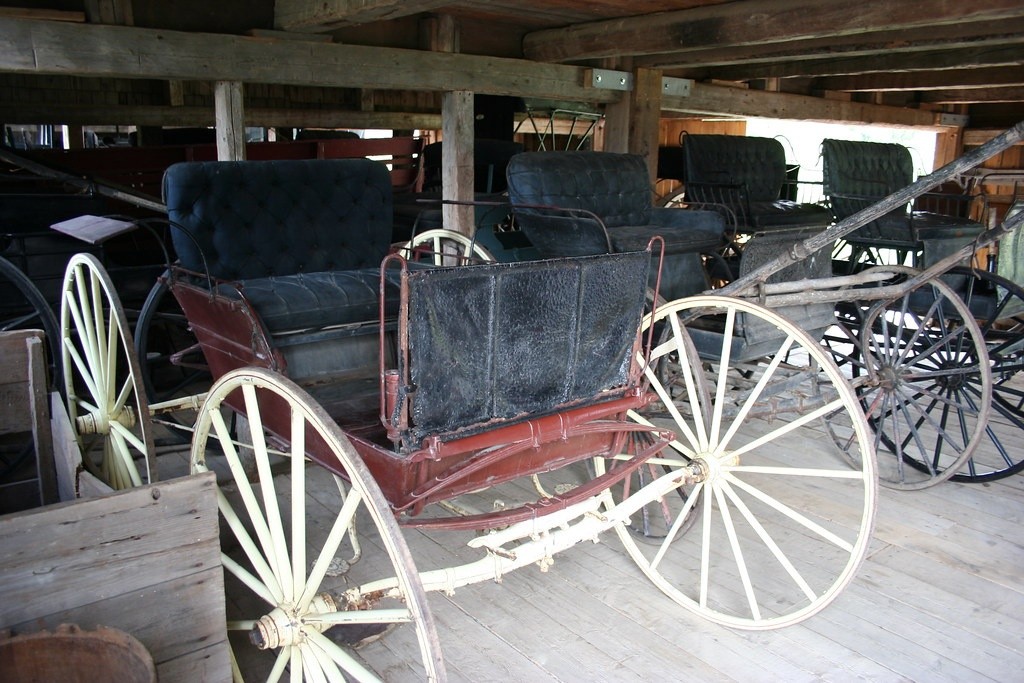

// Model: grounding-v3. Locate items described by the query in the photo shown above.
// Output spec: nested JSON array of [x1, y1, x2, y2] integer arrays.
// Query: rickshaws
[[55, 131, 1024, 682]]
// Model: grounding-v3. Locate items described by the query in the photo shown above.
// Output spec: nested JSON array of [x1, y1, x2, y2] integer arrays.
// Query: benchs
[[822, 138, 991, 244], [679, 135, 835, 233], [507, 153, 737, 256], [159, 156, 407, 401], [0, 174, 177, 318]]
